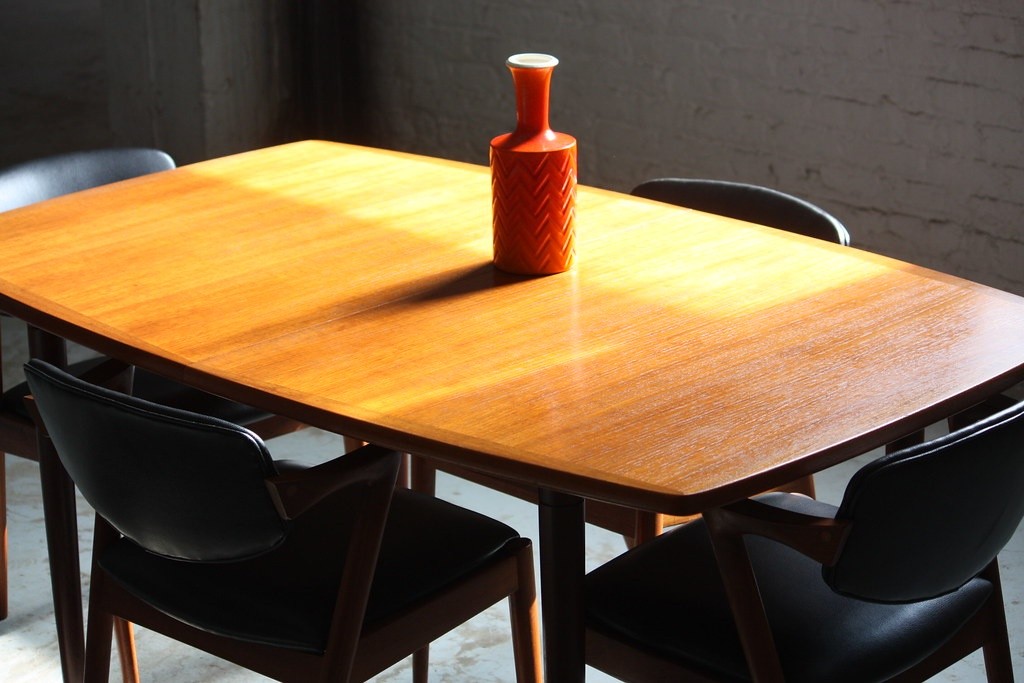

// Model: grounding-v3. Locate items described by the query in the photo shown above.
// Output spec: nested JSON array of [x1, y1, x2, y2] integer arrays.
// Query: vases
[[489, 52, 578, 274]]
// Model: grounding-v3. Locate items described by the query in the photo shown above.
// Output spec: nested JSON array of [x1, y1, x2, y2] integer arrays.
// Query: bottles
[[488, 51, 579, 273]]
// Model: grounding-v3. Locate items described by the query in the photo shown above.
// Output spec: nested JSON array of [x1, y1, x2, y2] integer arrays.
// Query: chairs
[[582, 395, 1024, 682], [0, 149, 407, 682], [413, 179, 853, 683], [23, 357, 542, 683]]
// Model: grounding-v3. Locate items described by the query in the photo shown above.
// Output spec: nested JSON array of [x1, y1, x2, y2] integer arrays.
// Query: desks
[[0, 138, 1024, 683]]
